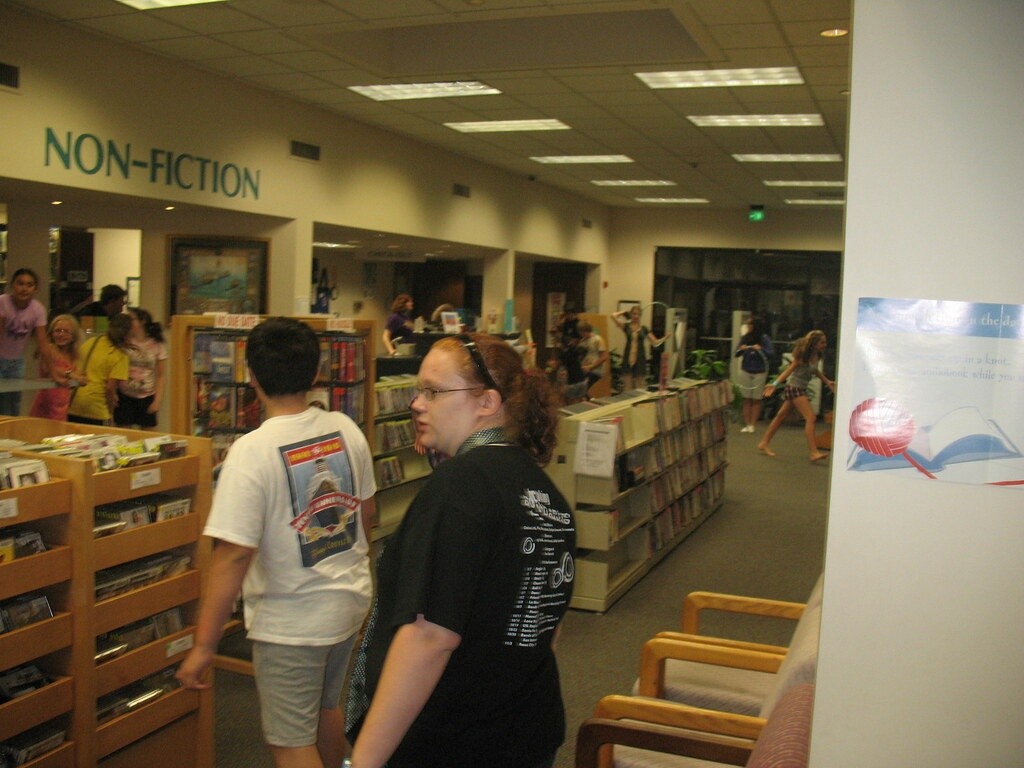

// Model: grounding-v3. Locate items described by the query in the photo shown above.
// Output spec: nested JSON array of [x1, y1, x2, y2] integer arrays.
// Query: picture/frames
[[171, 237, 270, 315]]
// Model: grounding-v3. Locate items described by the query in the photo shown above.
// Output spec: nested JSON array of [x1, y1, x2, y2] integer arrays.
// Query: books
[[372, 374, 428, 492], [0, 608, 182, 706], [1, 429, 190, 489], [194, 328, 369, 615], [557, 378, 732, 555], [0, 550, 190, 633], [0, 665, 185, 768], [0, 496, 193, 563]]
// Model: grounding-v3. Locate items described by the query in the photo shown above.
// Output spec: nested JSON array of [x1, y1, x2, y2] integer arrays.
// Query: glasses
[[414, 387, 505, 402], [51, 327, 73, 336]]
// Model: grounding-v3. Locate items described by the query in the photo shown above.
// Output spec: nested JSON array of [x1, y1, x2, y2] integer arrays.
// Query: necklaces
[[456, 426, 520, 457]]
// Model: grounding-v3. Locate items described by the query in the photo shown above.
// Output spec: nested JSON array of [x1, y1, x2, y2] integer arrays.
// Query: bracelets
[[341, 758, 353, 768], [772, 381, 779, 387], [65, 369, 72, 379]]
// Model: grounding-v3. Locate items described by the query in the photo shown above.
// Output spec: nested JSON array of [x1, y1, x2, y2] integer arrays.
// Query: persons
[[178, 316, 376, 768], [543, 305, 672, 406], [758, 330, 835, 463], [0, 266, 167, 431], [343, 332, 577, 768], [382, 294, 415, 356], [734, 315, 775, 434]]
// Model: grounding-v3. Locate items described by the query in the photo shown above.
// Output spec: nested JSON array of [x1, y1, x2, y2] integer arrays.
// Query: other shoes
[[741, 425, 754, 432]]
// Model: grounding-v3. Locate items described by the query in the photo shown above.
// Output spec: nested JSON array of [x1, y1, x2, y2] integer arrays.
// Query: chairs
[[574, 571, 823, 768]]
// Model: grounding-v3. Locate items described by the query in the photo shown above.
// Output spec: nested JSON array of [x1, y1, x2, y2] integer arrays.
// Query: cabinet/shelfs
[[1, 315, 732, 768]]
